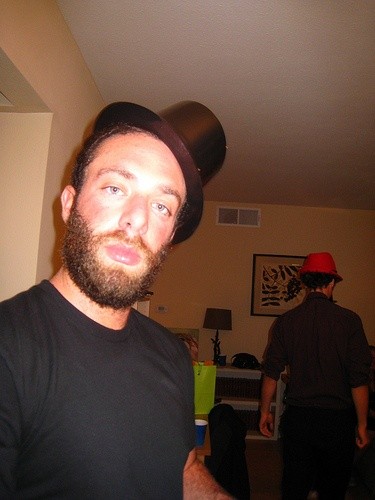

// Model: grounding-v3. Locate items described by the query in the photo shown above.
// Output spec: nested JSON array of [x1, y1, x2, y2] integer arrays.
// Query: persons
[[0, 121, 241, 500], [258, 253, 370, 500]]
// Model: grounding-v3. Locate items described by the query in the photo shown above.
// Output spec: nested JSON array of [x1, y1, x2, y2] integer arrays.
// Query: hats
[[92, 100, 227, 244], [297, 251, 343, 284]]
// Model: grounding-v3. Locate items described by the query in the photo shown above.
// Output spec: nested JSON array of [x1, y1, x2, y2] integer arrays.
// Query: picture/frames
[[250, 253, 333, 317]]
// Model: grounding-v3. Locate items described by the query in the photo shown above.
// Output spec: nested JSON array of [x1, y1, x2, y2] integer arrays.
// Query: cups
[[194, 419, 208, 446], [217, 354, 226, 367]]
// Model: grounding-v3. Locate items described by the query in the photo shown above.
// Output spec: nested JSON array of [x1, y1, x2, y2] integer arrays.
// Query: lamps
[[202, 307, 232, 365]]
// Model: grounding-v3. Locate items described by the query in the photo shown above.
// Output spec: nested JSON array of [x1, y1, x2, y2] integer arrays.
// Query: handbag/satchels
[[190, 358, 217, 415]]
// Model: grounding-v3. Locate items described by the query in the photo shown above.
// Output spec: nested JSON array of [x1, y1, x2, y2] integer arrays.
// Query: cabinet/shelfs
[[215, 365, 286, 441]]
